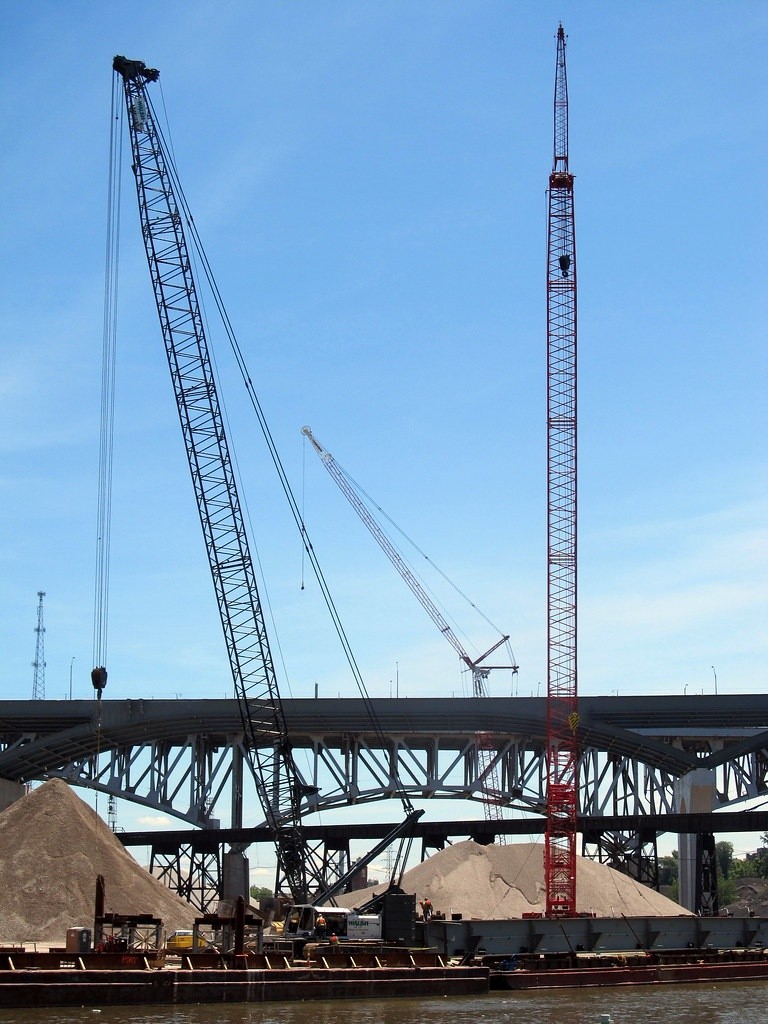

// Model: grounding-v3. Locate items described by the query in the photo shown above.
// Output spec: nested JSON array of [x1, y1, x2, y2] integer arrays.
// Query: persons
[[316, 914, 326, 940], [420, 898, 433, 921], [330, 932, 341, 953]]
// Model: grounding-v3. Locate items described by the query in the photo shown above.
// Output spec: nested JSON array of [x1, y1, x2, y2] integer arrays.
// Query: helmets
[[319, 914, 322, 917], [420, 902, 422, 904], [332, 933, 336, 936], [425, 897, 428, 900]]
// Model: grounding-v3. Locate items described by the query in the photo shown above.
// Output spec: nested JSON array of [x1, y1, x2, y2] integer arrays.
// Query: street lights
[[711, 665, 718, 696], [69, 656, 76, 701], [389, 680, 394, 699], [683, 684, 689, 697], [537, 682, 541, 699], [396, 662, 400, 698]]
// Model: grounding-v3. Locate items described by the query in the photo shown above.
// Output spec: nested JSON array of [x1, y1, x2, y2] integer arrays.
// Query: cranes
[[540, 18, 586, 914], [28, 590, 47, 700], [80, 49, 425, 907], [296, 422, 527, 841]]
